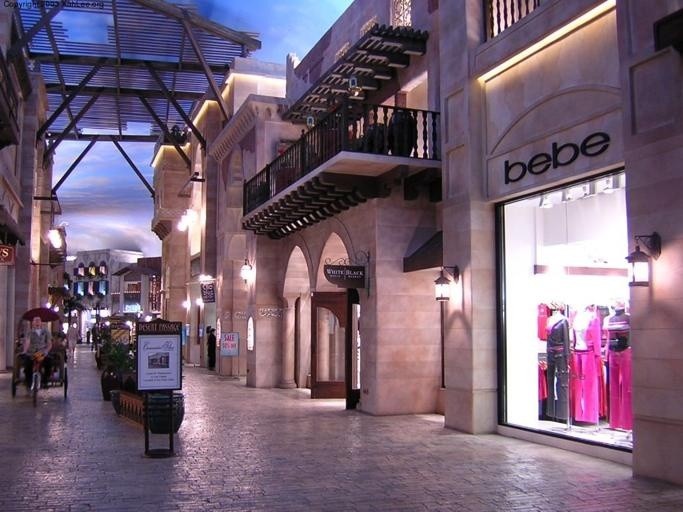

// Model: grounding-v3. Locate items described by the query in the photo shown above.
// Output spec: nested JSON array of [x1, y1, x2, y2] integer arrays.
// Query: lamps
[[626, 230, 661, 287], [434, 264, 460, 303]]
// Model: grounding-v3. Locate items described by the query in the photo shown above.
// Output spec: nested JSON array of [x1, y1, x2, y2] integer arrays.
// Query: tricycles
[[11, 308, 68, 407]]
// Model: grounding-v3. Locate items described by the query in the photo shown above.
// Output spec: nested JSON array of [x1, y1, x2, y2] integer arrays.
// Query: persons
[[605, 307, 635, 431], [67, 322, 81, 359], [571, 305, 605, 425], [21, 315, 54, 395], [90, 323, 101, 351], [209, 328, 216, 371], [543, 308, 570, 420]]
